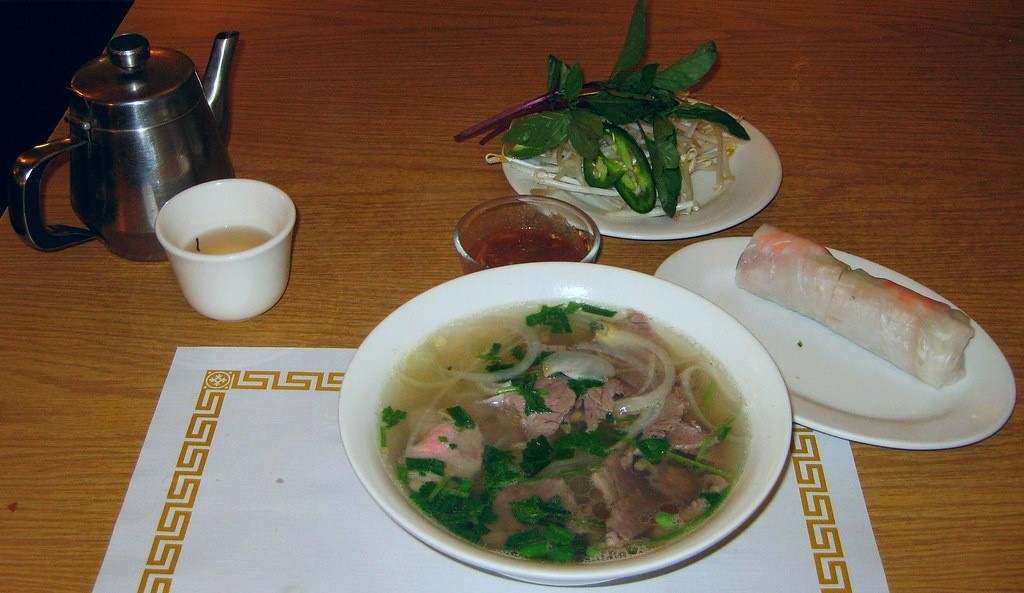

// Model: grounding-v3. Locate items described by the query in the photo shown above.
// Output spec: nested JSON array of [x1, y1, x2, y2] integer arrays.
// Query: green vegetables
[[379, 296, 742, 565], [454, 0, 751, 216]]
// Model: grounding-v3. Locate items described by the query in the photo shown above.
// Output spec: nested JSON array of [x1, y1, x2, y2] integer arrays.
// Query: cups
[[451, 192, 602, 275], [154, 179, 298, 321]]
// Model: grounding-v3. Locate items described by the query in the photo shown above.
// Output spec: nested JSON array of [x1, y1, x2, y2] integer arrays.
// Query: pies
[[732, 221, 974, 386]]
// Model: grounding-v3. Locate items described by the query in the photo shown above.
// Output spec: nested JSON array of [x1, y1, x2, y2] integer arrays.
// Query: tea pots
[[10, 30, 241, 262]]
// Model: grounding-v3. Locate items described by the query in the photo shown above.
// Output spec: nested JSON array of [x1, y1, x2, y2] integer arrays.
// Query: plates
[[502, 90, 783, 240], [653, 237, 1015, 451]]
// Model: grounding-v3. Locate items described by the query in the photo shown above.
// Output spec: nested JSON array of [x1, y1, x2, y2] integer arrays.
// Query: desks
[[0, 0, 1024, 593]]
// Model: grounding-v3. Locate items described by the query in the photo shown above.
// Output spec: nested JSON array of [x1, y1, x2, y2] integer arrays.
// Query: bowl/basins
[[339, 260, 793, 586]]
